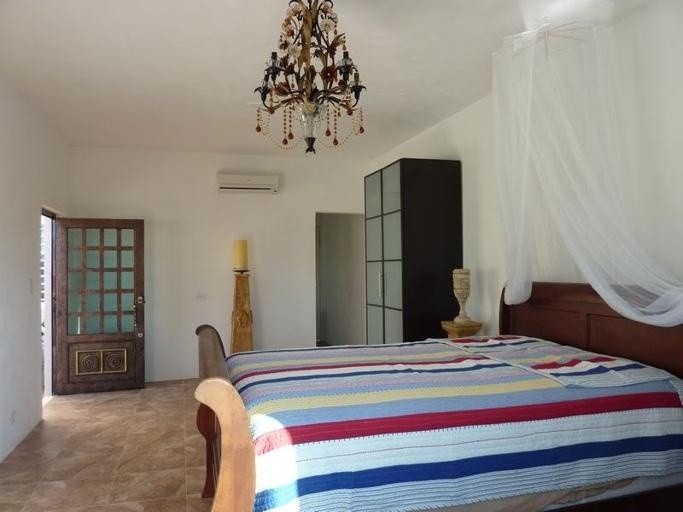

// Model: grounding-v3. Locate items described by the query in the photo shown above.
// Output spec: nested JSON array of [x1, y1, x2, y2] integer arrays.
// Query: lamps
[[452, 267, 472, 322], [252, 0, 366, 156]]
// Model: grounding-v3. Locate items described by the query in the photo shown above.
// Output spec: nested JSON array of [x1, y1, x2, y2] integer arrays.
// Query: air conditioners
[[215, 171, 280, 195]]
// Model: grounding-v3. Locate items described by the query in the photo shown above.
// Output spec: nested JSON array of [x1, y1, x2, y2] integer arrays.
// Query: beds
[[192, 280, 683, 512]]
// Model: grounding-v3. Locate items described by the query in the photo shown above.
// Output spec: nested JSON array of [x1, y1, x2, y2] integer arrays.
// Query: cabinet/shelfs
[[363, 157, 464, 346]]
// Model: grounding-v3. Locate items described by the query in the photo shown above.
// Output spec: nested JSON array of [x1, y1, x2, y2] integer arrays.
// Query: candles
[[234, 239, 249, 268]]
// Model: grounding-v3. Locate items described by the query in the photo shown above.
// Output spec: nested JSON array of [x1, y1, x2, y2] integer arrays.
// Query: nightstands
[[440, 320, 482, 339]]
